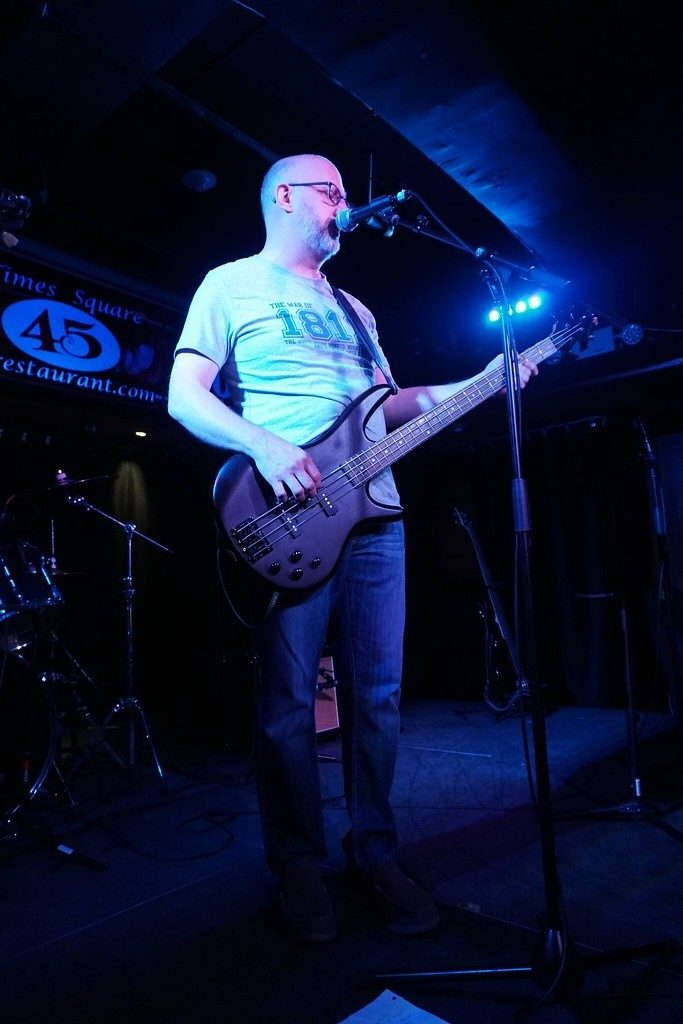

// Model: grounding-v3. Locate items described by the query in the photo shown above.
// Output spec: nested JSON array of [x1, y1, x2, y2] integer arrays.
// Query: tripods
[[360, 207, 682, 1023], [63, 488, 180, 797]]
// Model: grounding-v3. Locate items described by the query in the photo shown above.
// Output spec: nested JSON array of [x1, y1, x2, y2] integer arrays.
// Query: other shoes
[[277, 868, 336, 941], [343, 857, 440, 934]]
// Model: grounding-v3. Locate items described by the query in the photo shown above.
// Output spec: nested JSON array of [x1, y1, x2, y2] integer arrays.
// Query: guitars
[[208, 300, 603, 601]]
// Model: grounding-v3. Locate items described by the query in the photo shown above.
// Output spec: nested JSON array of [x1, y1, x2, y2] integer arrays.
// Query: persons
[[168, 154, 538, 938]]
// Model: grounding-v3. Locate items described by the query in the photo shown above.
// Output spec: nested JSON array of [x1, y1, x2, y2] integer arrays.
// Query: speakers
[[314, 646, 339, 738]]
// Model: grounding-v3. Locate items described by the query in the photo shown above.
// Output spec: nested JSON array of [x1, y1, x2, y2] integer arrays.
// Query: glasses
[[273, 181, 350, 209]]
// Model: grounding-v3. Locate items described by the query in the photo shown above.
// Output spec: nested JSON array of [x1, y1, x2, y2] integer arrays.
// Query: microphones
[[633, 417, 640, 428], [0, 501, 9, 521], [335, 191, 411, 232], [315, 680, 338, 693]]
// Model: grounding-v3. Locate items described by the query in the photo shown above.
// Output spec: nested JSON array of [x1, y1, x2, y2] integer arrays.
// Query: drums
[[0, 536, 68, 654], [0, 648, 61, 823]]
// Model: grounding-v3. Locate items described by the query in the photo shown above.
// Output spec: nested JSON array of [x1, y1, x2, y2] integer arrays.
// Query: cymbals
[[9, 463, 119, 505]]
